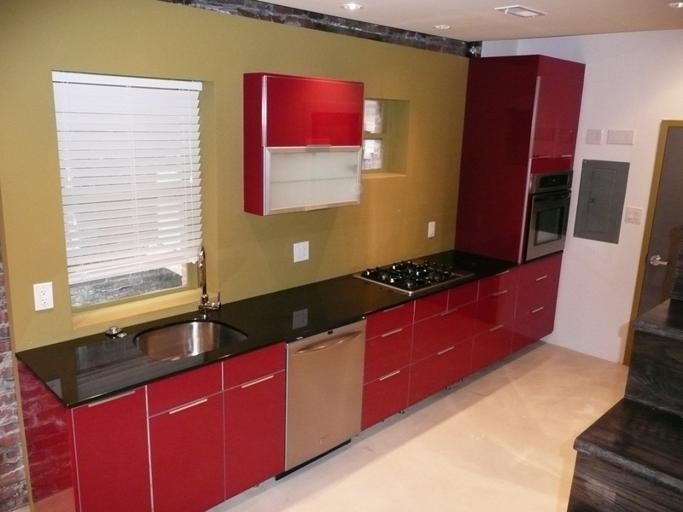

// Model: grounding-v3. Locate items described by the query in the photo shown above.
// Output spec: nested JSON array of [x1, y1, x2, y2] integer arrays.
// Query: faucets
[[198, 247, 208, 304]]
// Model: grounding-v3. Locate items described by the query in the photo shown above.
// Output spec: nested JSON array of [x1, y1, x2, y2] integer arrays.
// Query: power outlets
[[32, 281, 54, 313]]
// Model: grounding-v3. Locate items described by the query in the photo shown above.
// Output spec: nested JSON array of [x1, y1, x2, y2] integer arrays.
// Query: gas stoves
[[352, 258, 465, 297]]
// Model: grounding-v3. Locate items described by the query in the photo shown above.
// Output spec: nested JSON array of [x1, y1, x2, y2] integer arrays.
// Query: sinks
[[134, 322, 247, 362]]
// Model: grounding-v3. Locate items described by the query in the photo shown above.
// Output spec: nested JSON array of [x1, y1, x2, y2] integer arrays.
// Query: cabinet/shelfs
[[142, 344, 285, 509], [519, 251, 561, 360], [11, 362, 153, 510], [413, 277, 471, 418], [471, 269, 517, 378], [242, 70, 366, 216], [362, 303, 412, 436]]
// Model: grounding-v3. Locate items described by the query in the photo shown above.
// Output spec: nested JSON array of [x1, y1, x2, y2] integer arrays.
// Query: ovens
[[520, 171, 571, 261]]
